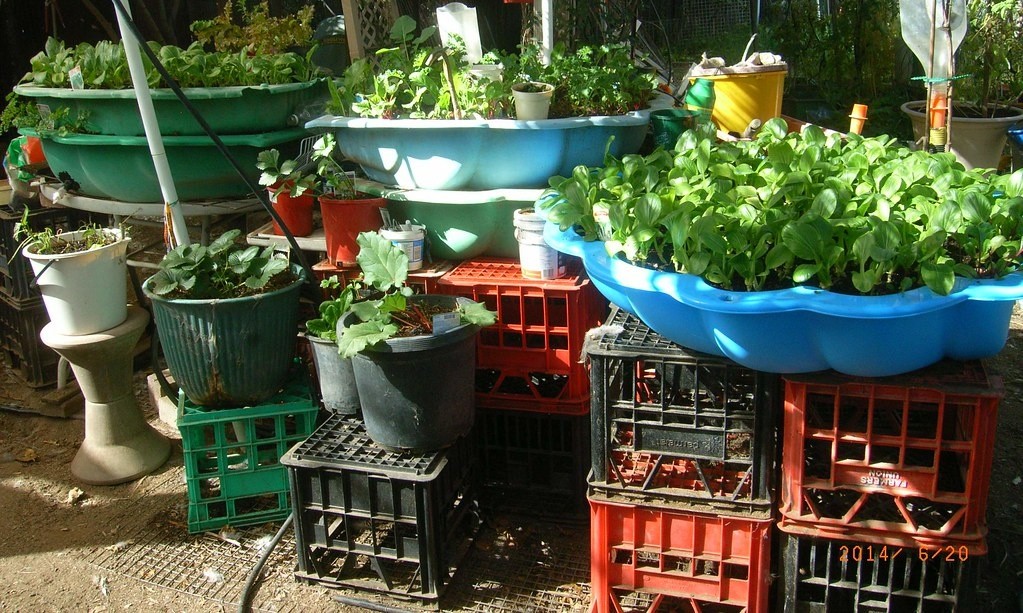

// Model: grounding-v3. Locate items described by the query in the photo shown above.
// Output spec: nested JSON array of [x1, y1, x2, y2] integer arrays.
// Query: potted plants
[[1, 0, 1022, 455]]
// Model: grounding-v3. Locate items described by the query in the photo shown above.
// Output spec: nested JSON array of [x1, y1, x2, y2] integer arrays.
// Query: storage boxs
[[436, 249, 614, 494], [173, 380, 325, 537], [577, 296, 1007, 612], [285, 402, 492, 605]]
